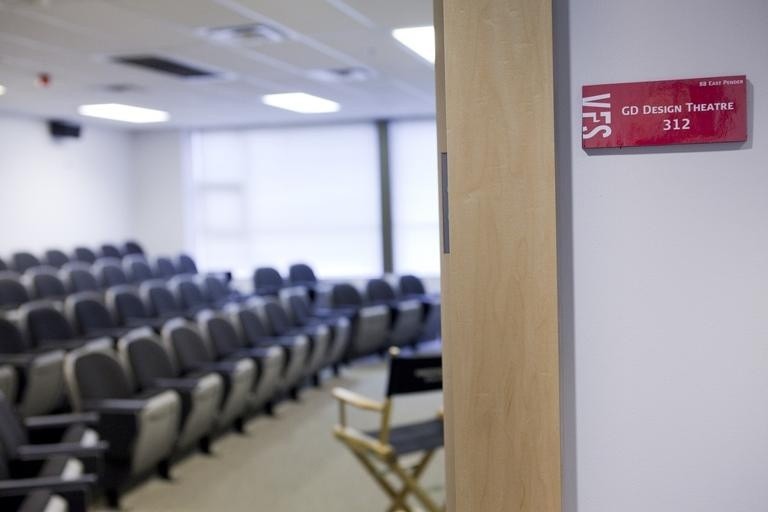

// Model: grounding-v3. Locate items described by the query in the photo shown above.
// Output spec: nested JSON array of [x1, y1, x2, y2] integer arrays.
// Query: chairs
[[0, 241, 446, 512]]
